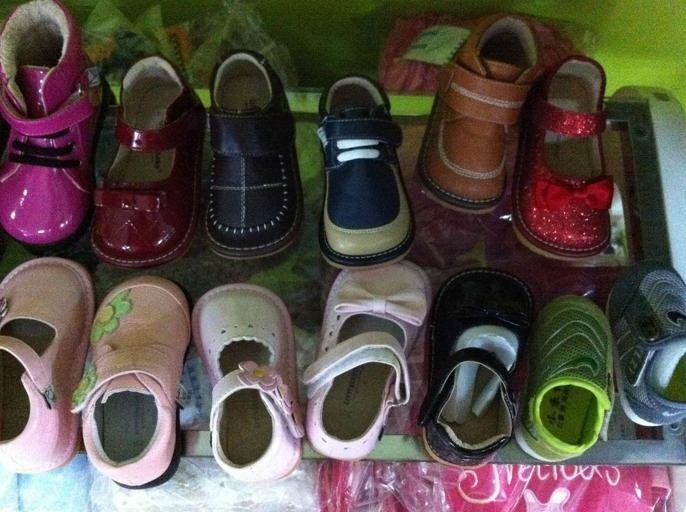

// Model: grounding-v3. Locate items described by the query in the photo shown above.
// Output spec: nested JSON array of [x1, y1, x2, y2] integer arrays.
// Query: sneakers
[[512, 293, 615, 462], [598, 258, 686, 442]]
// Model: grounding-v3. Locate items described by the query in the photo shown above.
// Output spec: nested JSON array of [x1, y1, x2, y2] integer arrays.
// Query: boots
[[0, 1, 113, 255]]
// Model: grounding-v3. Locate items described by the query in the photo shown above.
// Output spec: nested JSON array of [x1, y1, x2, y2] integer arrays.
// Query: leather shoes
[[513, 54, 615, 261], [302, 259, 434, 461], [416, 13, 544, 216], [67, 274, 191, 491], [205, 48, 304, 261], [89, 55, 209, 270], [314, 73, 418, 269], [416, 267, 535, 469], [190, 282, 305, 485], [0, 256, 95, 475]]
